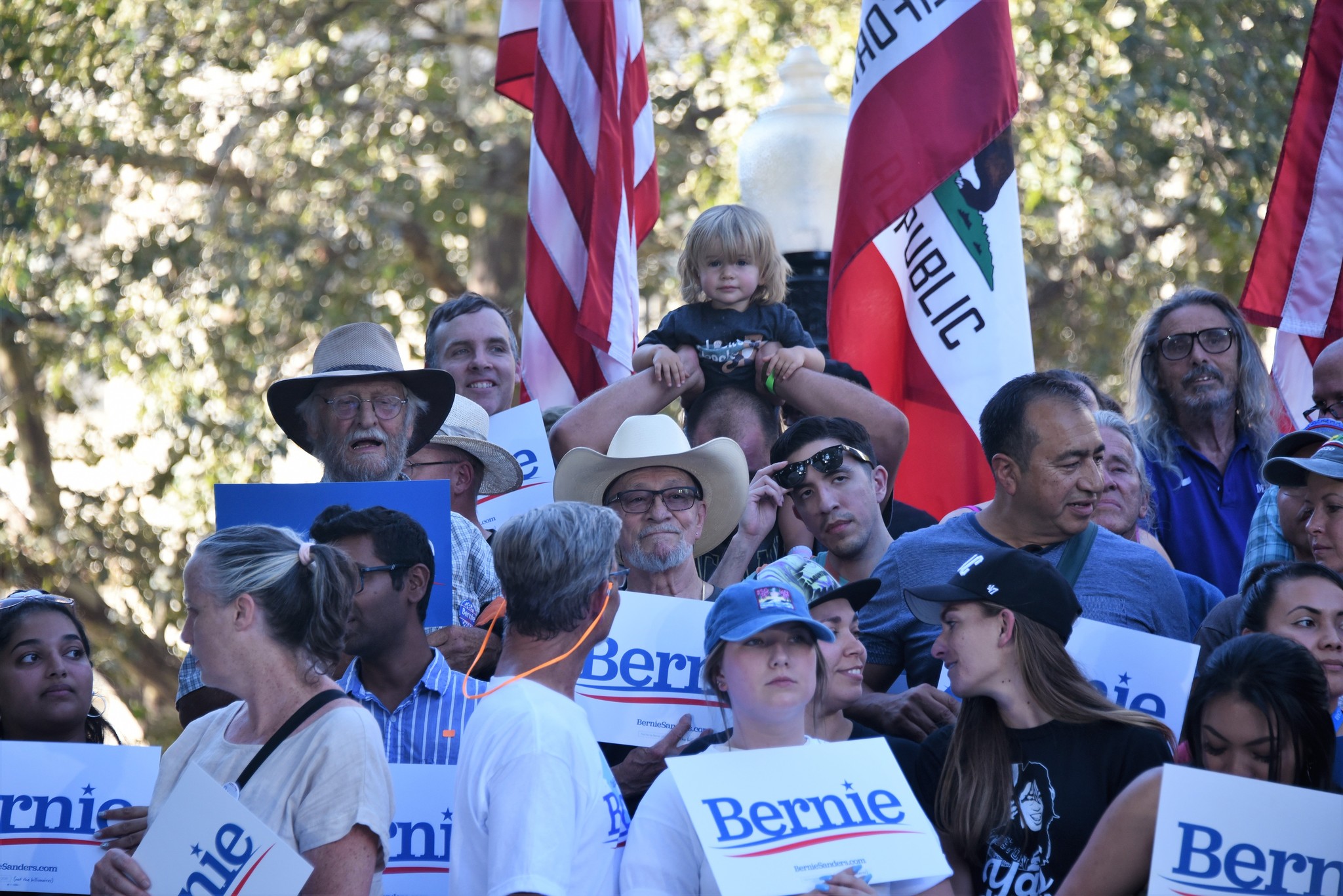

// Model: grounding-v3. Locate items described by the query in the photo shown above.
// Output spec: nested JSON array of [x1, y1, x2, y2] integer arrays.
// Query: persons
[[308, 505, 489, 896], [552, 413, 751, 821], [633, 204, 826, 410], [176, 322, 507, 730], [902, 550, 1174, 896], [1031, 562, 1343, 896], [401, 286, 1343, 684], [858, 373, 1193, 746], [89, 524, 390, 896], [618, 555, 973, 896], [450, 501, 633, 896], [0, 588, 148, 896]]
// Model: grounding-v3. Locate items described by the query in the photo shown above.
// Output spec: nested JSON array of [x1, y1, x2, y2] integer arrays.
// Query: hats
[[430, 392, 525, 497], [266, 322, 459, 461], [1261, 433, 1343, 487], [755, 552, 883, 615], [901, 547, 1083, 645], [549, 414, 751, 563], [702, 580, 836, 664], [1265, 416, 1343, 460]]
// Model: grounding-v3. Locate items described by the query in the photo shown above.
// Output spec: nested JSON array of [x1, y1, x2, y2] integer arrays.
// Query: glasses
[[1302, 400, 1343, 424], [400, 456, 460, 476], [1150, 327, 1235, 361], [354, 564, 411, 595], [317, 391, 410, 420], [772, 442, 875, 490], [607, 486, 701, 514], [0, 593, 78, 618]]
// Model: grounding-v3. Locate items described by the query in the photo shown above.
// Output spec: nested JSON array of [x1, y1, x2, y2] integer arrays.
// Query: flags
[[492, 0, 662, 416], [1237, 0, 1343, 439], [826, 0, 1035, 523]]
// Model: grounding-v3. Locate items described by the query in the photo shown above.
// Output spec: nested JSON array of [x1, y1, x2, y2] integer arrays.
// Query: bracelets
[[765, 368, 775, 396]]
